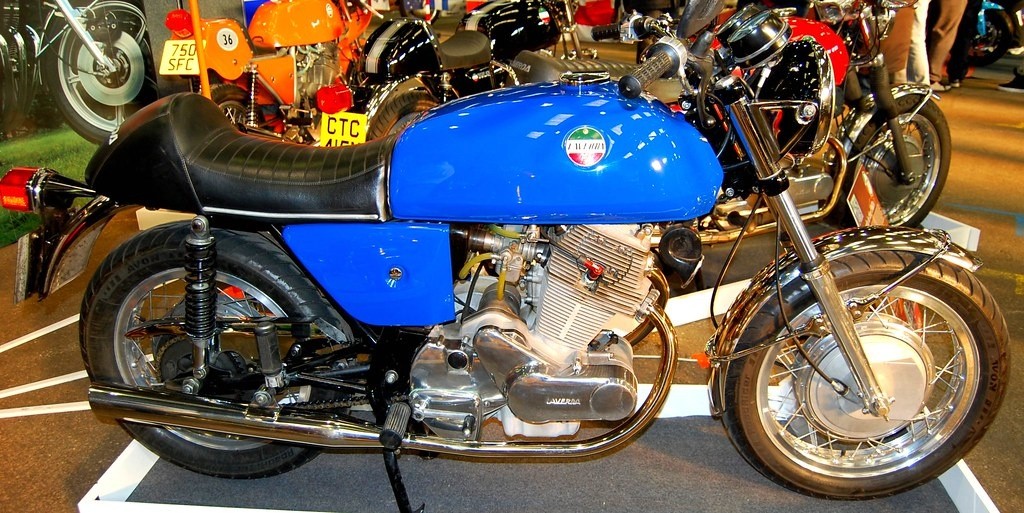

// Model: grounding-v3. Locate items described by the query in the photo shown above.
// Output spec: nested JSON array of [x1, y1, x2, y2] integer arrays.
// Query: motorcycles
[[0, 0, 1024, 513]]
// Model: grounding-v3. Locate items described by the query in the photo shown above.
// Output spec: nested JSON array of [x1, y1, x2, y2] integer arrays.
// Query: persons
[[867, 0, 1024, 93], [613, 0, 672, 63]]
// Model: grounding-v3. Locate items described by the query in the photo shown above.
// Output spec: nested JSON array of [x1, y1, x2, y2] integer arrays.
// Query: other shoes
[[945, 85, 951, 90], [997, 76, 1024, 92], [930, 82, 945, 91], [950, 83, 960, 87]]
